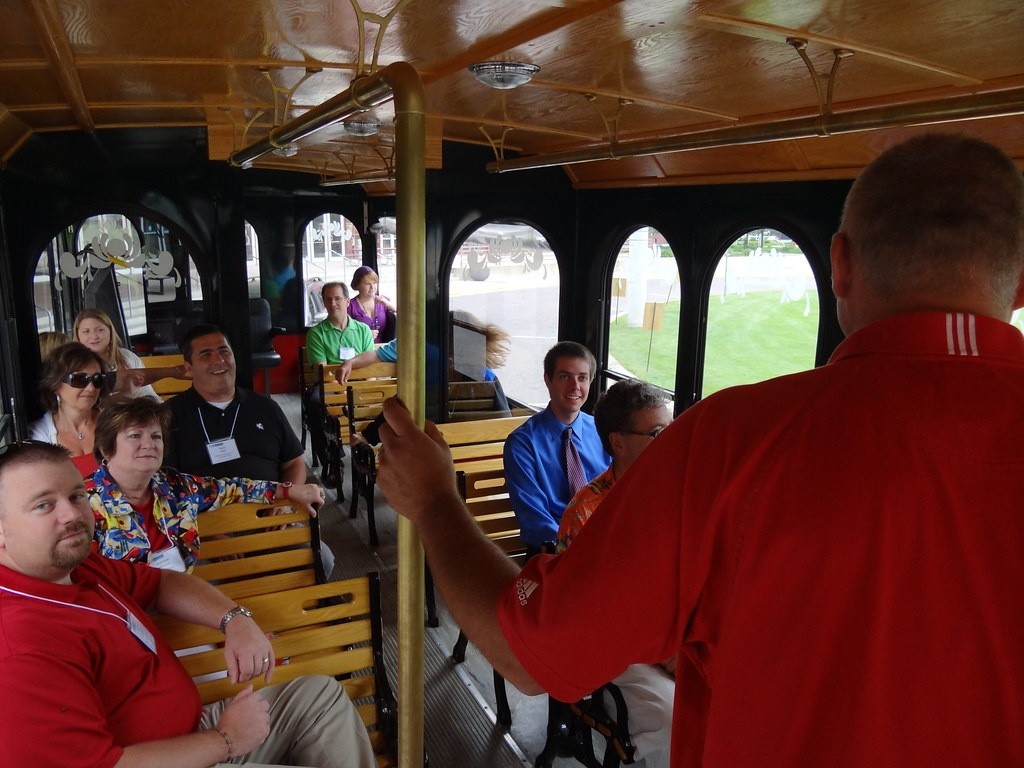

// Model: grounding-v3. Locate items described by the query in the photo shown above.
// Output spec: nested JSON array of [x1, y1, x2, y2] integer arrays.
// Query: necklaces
[[78, 434, 84, 439]]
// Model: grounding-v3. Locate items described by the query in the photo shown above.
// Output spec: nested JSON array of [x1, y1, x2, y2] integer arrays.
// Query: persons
[[351, 311, 511, 447], [81, 400, 325, 683], [347, 266, 397, 343], [40, 333, 193, 391], [303, 282, 374, 487], [0, 440, 377, 768], [74, 310, 164, 404], [335, 338, 398, 386], [161, 326, 336, 580], [25, 340, 106, 480], [502, 341, 613, 549], [374, 134, 1024, 768], [554, 379, 678, 768]]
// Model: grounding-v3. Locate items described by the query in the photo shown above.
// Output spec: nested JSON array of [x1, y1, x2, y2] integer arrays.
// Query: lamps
[[341, 120, 385, 137], [271, 145, 302, 158], [467, 61, 541, 89]]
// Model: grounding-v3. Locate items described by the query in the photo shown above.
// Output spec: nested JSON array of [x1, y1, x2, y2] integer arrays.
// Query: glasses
[[617, 425, 667, 439], [62, 371, 107, 389]]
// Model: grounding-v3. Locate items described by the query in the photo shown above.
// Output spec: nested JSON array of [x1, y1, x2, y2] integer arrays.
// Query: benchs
[[296, 343, 388, 468], [194, 499, 354, 681], [149, 570, 429, 768], [450, 469, 529, 728], [346, 381, 499, 553], [532, 539, 637, 768], [140, 354, 192, 401], [318, 361, 397, 503]]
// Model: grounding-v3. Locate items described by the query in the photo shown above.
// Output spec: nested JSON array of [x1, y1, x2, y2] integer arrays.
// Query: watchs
[[220, 606, 252, 634], [281, 482, 292, 499]]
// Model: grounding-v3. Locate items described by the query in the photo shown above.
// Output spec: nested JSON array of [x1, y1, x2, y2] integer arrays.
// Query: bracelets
[[215, 728, 234, 753]]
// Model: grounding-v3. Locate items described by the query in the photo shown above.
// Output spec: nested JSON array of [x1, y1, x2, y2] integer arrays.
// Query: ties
[[563, 429, 588, 499]]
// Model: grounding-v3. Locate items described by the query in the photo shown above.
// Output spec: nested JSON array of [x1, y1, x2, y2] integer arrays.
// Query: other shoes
[[325, 461, 344, 489]]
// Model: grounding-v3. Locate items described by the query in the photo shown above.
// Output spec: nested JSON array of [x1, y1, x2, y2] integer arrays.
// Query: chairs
[[248, 298, 287, 397]]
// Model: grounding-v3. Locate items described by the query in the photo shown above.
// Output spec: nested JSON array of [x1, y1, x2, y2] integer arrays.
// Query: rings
[[263, 658, 268, 663]]
